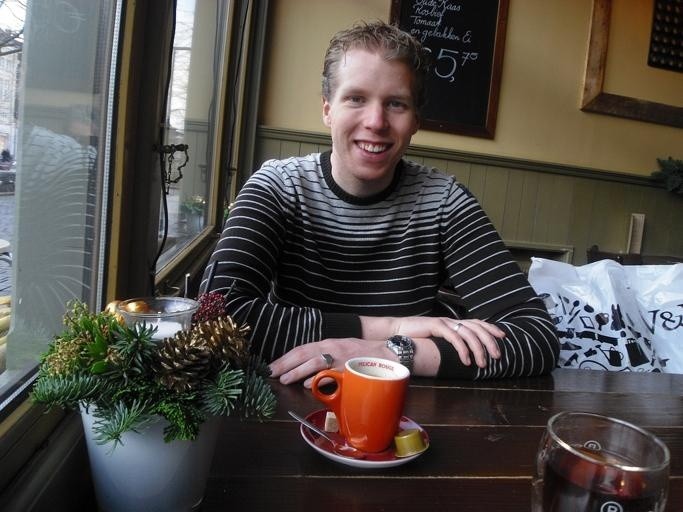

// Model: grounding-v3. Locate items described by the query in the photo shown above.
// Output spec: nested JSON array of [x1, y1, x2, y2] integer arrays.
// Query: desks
[[44, 354, 683, 511]]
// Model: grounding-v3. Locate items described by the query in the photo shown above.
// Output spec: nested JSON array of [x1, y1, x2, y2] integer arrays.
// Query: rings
[[454, 323, 462, 332], [321, 353, 334, 369]]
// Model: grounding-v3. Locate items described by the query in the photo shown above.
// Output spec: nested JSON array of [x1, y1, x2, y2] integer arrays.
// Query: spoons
[[287, 408, 371, 461]]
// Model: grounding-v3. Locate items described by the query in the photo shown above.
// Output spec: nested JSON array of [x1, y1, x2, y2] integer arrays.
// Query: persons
[[196, 19, 559, 391], [12, 118, 95, 351]]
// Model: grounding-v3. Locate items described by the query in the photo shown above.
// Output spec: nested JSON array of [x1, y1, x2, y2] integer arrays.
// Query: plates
[[302, 408, 429, 468]]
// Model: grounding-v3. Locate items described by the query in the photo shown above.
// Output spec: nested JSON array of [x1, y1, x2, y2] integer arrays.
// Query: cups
[[116, 296, 199, 343], [528, 409, 671, 512], [313, 356, 411, 452]]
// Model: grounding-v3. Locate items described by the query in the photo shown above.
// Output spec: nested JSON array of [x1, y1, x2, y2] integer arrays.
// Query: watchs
[[387, 335, 416, 386]]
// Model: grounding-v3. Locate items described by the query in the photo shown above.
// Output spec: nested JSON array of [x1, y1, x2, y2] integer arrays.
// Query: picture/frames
[[574, 1, 682, 131]]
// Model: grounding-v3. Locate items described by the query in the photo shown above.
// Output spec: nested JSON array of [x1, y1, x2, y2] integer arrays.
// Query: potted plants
[[22, 290, 280, 512], [179, 192, 207, 233]]
